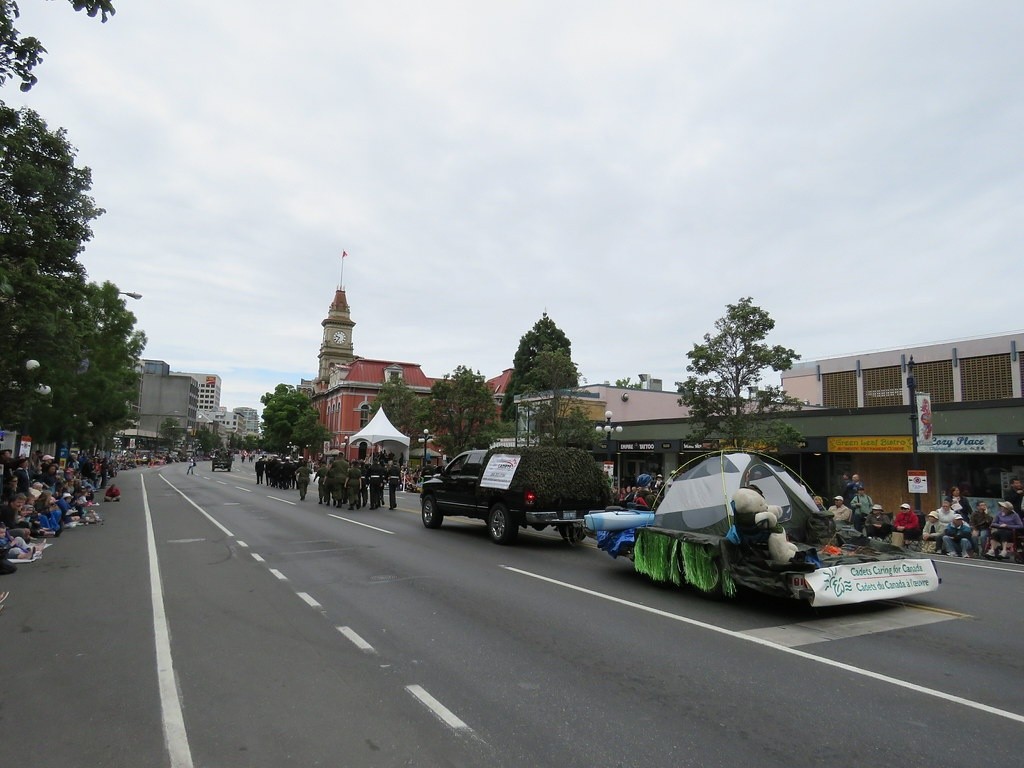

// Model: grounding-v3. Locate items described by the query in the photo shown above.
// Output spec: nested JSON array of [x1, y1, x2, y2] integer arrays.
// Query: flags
[[343, 251, 348, 257]]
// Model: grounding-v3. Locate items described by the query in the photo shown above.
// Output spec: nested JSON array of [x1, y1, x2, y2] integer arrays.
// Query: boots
[[319, 498, 397, 510]]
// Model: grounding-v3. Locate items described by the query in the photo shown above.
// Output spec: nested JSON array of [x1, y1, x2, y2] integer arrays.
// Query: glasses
[[901, 507, 909, 510]]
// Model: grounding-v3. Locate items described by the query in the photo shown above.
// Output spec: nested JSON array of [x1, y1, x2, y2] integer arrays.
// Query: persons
[[812, 472, 1024, 560], [0, 444, 446, 613], [607, 469, 676, 510]]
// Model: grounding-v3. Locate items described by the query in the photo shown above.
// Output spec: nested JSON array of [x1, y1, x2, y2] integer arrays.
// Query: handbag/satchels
[[837, 529, 869, 546]]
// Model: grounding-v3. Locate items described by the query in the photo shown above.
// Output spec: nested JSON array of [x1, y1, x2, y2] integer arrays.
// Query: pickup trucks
[[419, 447, 619, 546]]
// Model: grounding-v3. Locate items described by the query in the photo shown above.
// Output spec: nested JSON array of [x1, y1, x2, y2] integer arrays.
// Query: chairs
[[875, 510, 926, 553]]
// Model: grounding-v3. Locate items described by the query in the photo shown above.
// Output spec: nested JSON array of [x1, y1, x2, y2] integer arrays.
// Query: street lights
[[341, 435, 349, 459], [155, 410, 179, 456], [417, 428, 434, 465], [595, 411, 624, 462], [287, 442, 294, 455], [8, 359, 52, 459]]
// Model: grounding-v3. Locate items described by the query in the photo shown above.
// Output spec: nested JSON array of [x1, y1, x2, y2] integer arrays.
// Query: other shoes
[[986, 550, 995, 558], [37, 538, 46, 551], [964, 553, 969, 558], [947, 551, 958, 557], [999, 550, 1007, 558], [936, 549, 942, 555], [27, 546, 36, 560], [973, 552, 986, 559], [905, 544, 911, 551]]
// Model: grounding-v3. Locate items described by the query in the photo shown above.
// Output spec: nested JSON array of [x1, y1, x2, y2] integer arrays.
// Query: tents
[[348, 406, 410, 493]]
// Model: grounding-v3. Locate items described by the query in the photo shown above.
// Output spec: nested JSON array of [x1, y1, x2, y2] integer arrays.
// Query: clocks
[[332, 330, 347, 344]]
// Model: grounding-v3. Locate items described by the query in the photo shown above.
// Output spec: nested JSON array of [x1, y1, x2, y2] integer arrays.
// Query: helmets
[[637, 474, 652, 487]]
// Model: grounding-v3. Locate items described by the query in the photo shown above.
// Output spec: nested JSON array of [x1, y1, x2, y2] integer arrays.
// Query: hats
[[900, 503, 910, 510], [857, 486, 865, 491], [954, 514, 964, 519], [656, 474, 662, 478], [62, 493, 74, 499], [998, 501, 1015, 514], [42, 455, 55, 460], [833, 495, 844, 501], [670, 470, 675, 476], [51, 496, 59, 502], [872, 504, 884, 513], [928, 511, 939, 520]]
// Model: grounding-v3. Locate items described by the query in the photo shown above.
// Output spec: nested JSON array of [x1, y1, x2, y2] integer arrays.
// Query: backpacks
[[807, 510, 838, 546]]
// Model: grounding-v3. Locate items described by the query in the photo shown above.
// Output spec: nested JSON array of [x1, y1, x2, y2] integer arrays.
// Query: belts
[[391, 476, 399, 478], [371, 475, 380, 477], [361, 476, 365, 478]]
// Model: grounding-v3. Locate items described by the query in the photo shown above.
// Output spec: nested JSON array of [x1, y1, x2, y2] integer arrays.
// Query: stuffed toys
[[735, 485, 799, 563]]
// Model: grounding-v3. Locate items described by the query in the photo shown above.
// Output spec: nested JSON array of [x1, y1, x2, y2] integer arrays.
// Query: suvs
[[211, 448, 235, 473]]
